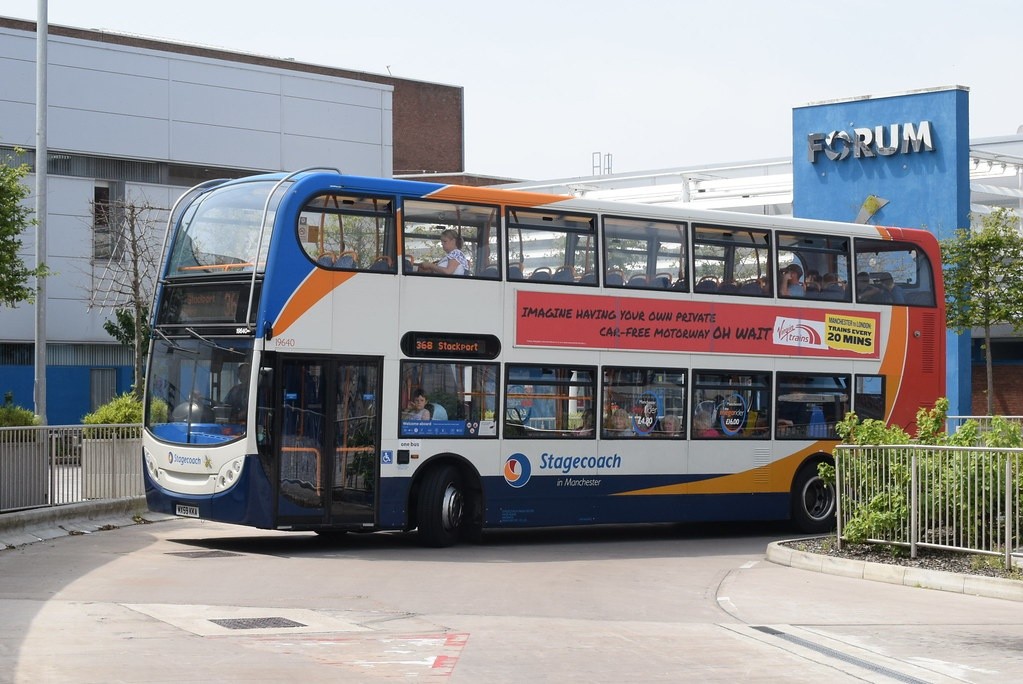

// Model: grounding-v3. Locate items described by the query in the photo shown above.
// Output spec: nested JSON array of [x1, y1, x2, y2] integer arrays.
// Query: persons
[[222, 363, 264, 424], [402, 389, 431, 421], [420, 230, 468, 275], [580, 377, 806, 439], [758, 263, 907, 304]]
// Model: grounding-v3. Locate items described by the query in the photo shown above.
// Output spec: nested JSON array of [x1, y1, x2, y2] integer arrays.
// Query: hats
[[780, 264, 803, 277]]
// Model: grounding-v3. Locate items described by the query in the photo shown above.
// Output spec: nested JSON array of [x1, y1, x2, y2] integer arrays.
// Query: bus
[[141, 166, 947, 547]]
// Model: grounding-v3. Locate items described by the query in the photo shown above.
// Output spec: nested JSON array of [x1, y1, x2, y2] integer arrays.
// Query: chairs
[[319, 250, 847, 303]]
